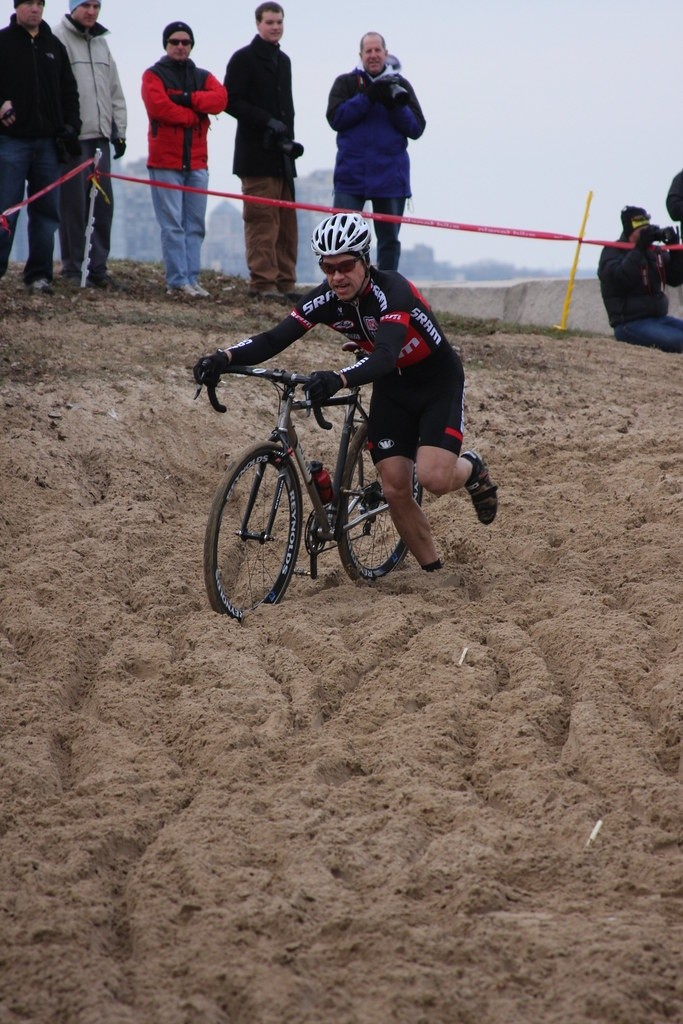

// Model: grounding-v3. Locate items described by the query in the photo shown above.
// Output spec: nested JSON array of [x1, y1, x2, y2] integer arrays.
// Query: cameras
[[268, 131, 304, 160], [649, 226, 675, 244], [380, 75, 410, 106]]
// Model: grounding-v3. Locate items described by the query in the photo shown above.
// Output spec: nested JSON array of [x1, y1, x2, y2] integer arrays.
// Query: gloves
[[368, 77, 397, 107], [638, 226, 680, 246], [193, 349, 229, 388], [269, 120, 287, 139], [302, 369, 345, 401], [112, 139, 129, 158]]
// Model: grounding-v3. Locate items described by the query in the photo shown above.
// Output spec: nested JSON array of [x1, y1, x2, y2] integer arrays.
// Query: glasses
[[317, 251, 360, 273], [168, 37, 192, 46]]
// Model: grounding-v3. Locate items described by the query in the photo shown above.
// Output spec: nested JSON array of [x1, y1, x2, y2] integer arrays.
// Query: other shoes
[[248, 280, 303, 302], [167, 282, 211, 299], [24, 278, 52, 294]]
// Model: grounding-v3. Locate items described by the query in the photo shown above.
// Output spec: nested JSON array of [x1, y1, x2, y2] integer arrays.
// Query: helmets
[[310, 212, 370, 258]]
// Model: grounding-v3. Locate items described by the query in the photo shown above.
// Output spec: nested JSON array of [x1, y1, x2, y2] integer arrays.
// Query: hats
[[163, 21, 195, 49], [69, 0, 101, 12], [621, 206, 650, 230]]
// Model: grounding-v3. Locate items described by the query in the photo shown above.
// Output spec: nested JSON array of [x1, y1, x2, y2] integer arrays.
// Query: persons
[[192, 212, 500, 575], [666, 168, 683, 242], [140, 22, 229, 302], [55, 0, 128, 295], [0, 0, 83, 297], [597, 205, 683, 353], [222, 1, 305, 298], [326, 32, 427, 270]]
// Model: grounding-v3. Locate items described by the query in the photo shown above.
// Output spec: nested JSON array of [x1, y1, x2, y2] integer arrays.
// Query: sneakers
[[460, 450, 498, 525]]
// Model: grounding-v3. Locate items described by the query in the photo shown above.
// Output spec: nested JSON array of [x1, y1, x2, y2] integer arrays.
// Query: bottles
[[310, 461, 333, 504]]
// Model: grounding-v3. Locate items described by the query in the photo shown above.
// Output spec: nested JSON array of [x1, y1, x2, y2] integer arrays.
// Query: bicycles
[[192, 339, 425, 623]]
